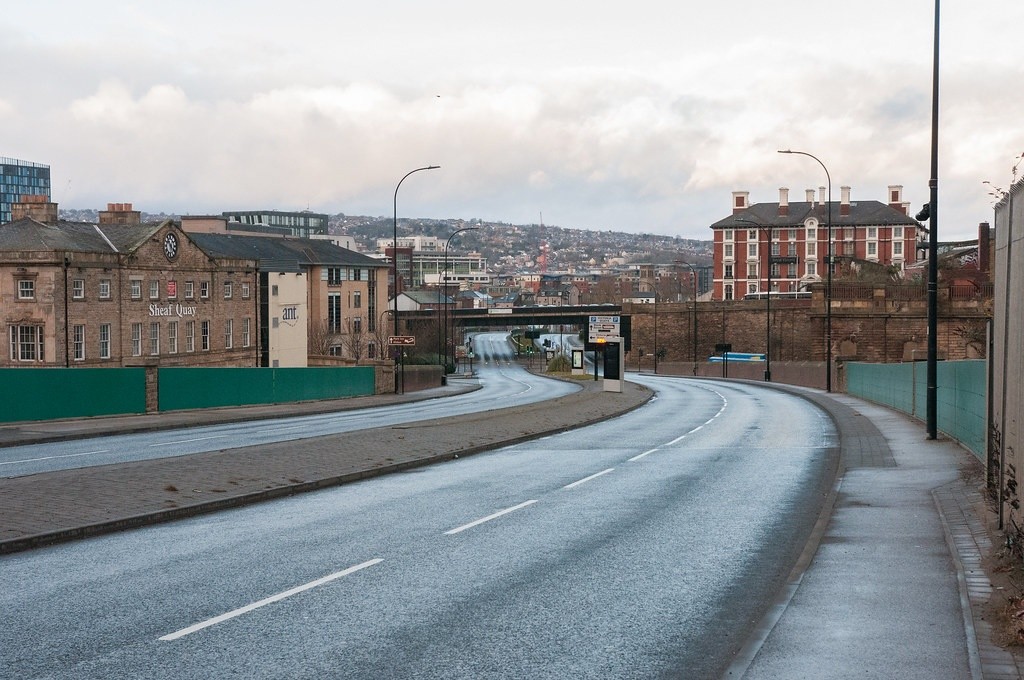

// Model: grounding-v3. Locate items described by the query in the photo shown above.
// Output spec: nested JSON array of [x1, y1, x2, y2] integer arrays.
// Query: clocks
[[163, 231, 180, 263]]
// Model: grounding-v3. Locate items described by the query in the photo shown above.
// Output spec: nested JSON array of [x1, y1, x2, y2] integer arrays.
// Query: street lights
[[674, 260, 697, 369], [394, 165, 441, 393], [733, 218, 770, 380], [778, 149, 832, 391], [438, 263, 463, 363], [636, 281, 657, 372], [445, 227, 480, 372]]
